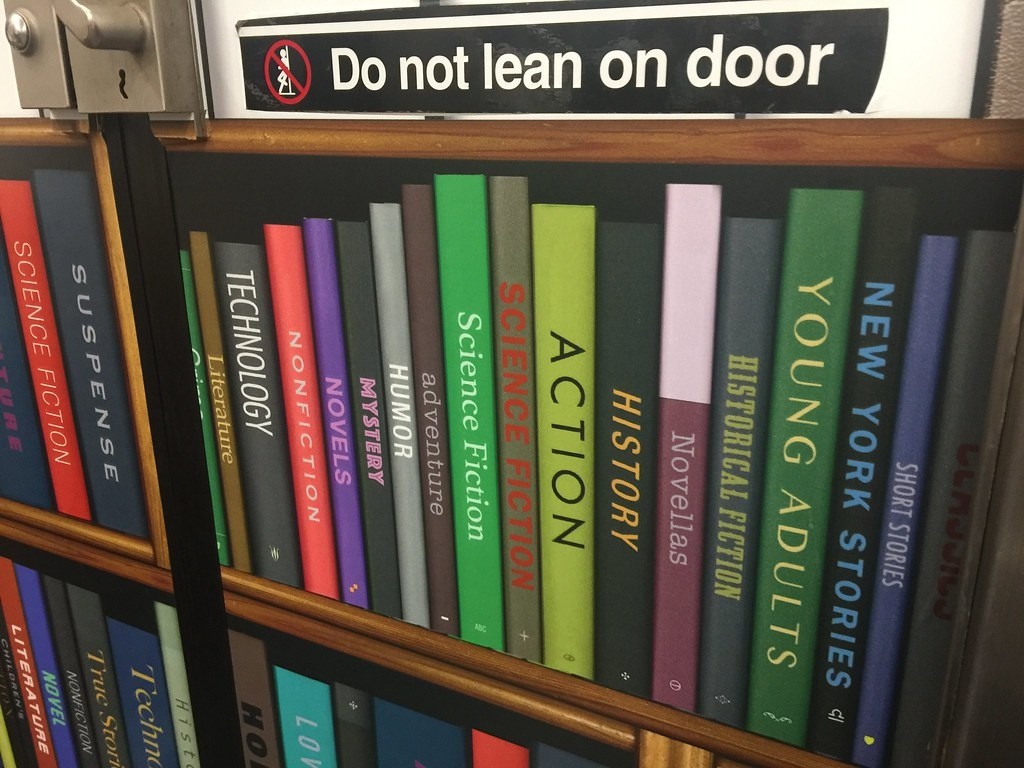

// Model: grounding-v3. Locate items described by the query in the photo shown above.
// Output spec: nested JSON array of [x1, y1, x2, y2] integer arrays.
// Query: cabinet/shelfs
[[0, 116, 1024, 768]]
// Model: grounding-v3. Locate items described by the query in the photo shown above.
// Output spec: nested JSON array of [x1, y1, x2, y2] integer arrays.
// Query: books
[[181, 169, 1023, 767], [0, 157, 195, 768]]
